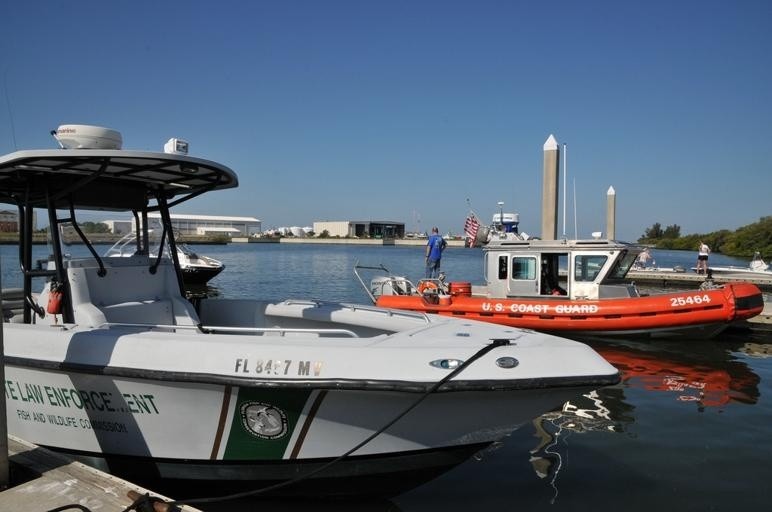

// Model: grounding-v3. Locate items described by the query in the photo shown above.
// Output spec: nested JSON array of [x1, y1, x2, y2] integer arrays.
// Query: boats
[[354, 143, 766, 340], [33, 224, 225, 287], [0, 124, 621, 462], [480, 202, 771, 292]]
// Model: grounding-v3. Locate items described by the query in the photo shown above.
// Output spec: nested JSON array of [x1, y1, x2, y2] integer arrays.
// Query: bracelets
[[425, 255, 429, 257]]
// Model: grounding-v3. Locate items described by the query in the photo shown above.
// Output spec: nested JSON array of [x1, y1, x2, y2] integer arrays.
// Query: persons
[[637, 248, 653, 268], [425, 225, 448, 285], [696, 240, 711, 274]]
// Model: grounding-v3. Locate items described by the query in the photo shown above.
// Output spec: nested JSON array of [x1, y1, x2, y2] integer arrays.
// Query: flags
[[464, 214, 480, 250]]
